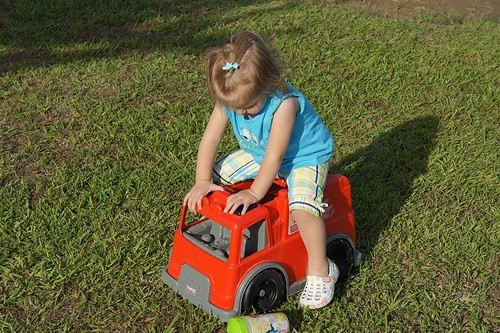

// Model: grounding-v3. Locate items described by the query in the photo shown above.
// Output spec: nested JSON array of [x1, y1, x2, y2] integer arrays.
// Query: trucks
[[160, 172, 362, 323]]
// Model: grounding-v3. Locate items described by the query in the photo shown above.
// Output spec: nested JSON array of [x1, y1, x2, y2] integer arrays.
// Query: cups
[[227, 312, 289, 333]]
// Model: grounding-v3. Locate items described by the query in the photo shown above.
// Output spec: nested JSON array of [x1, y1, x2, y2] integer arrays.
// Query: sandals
[[300, 257, 339, 309]]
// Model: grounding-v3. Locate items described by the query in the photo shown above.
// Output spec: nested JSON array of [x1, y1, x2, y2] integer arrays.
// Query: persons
[[182, 29, 340, 310]]
